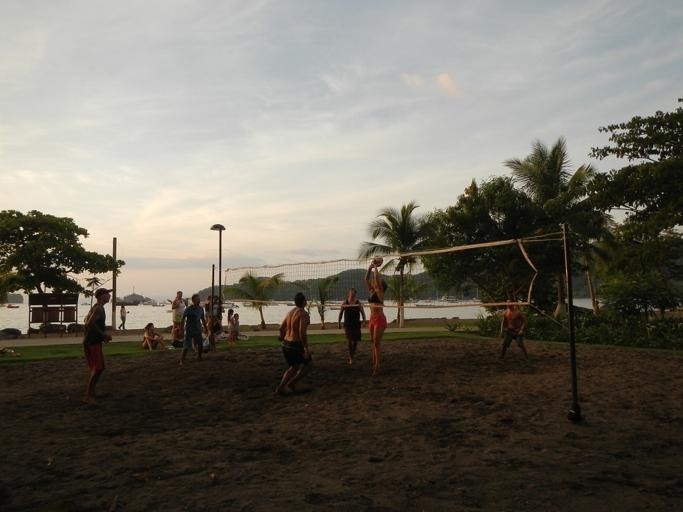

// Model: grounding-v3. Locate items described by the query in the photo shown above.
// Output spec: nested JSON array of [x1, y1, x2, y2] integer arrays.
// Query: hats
[[95, 288, 113, 298]]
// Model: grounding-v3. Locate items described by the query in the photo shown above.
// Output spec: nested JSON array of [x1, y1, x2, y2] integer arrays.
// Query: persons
[[83, 288, 114, 408], [141, 323, 165, 353], [118, 304, 130, 331], [276, 292, 311, 394], [498, 299, 531, 360], [364, 261, 387, 378], [337, 287, 366, 365], [167, 290, 240, 368]]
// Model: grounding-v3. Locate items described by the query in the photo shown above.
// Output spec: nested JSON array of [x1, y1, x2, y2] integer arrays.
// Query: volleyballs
[[373, 255, 383, 267]]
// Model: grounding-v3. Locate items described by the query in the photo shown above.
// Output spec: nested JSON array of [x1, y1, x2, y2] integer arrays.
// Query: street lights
[[208, 221, 227, 332]]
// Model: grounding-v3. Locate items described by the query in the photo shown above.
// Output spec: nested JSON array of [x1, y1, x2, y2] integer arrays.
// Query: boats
[[6, 303, 19, 308], [414, 294, 463, 310], [112, 298, 167, 309]]
